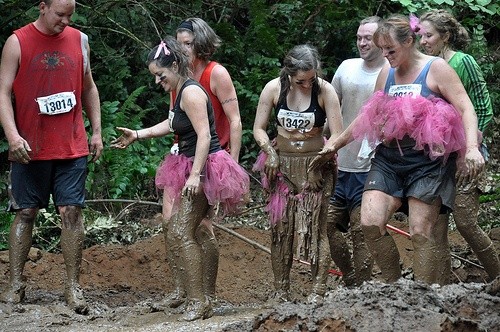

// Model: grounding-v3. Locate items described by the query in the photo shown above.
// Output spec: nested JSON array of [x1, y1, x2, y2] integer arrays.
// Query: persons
[[109, 15, 252, 323], [254, 44, 344, 308], [305, 9, 500, 287], [0, 0, 103, 314]]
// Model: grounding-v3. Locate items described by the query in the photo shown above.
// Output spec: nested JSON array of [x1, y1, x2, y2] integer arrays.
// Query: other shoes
[[179, 297, 213, 321], [64, 282, 89, 315], [151, 294, 184, 312], [264, 291, 287, 308], [303, 292, 324, 309], [0, 282, 25, 304]]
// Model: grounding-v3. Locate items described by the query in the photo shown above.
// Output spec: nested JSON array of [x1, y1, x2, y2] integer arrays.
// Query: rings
[[188, 189, 191, 191]]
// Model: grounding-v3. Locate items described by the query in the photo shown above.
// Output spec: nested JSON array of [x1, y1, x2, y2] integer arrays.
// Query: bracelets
[[136, 130, 138, 140]]
